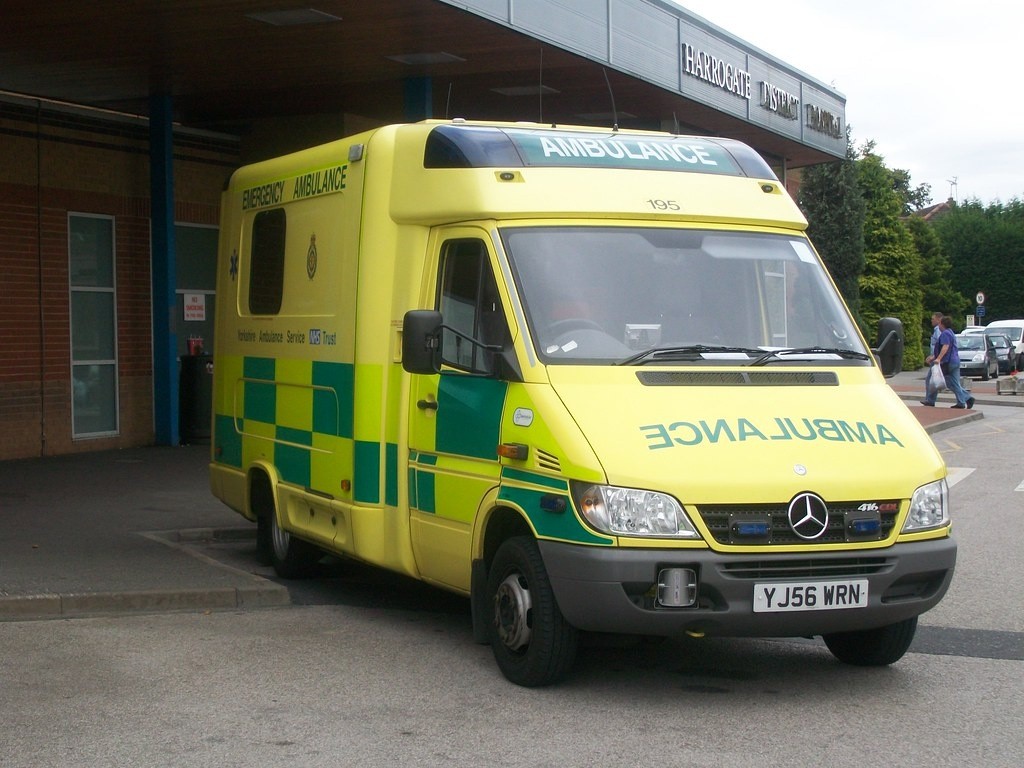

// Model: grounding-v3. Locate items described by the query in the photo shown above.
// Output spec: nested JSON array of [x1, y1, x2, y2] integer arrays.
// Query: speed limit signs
[[976, 291, 985, 305]]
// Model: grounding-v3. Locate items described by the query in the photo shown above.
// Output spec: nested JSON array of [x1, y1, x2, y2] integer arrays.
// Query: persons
[[920, 312, 975, 409]]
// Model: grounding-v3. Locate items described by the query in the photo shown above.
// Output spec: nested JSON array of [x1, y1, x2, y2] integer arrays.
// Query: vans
[[959, 326, 987, 334], [983, 319, 1024, 373]]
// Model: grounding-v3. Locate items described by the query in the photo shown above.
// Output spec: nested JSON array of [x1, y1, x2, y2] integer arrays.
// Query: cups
[[187, 339, 196, 356], [196, 338, 204, 355]]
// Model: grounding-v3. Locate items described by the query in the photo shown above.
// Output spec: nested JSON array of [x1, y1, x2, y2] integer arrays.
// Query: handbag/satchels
[[931, 360, 946, 390]]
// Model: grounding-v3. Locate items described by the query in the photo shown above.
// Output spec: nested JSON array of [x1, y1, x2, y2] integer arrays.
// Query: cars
[[986, 332, 1016, 375], [954, 333, 999, 381]]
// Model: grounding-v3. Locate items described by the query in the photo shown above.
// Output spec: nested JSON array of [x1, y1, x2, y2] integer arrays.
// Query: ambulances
[[208, 117, 958, 690]]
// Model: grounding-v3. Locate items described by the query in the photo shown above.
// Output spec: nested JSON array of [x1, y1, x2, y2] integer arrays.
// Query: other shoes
[[921, 401, 927, 405], [951, 404, 965, 408], [966, 397, 975, 409]]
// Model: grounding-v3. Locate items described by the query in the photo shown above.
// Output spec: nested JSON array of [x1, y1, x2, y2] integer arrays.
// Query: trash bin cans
[[179, 354, 213, 446]]
